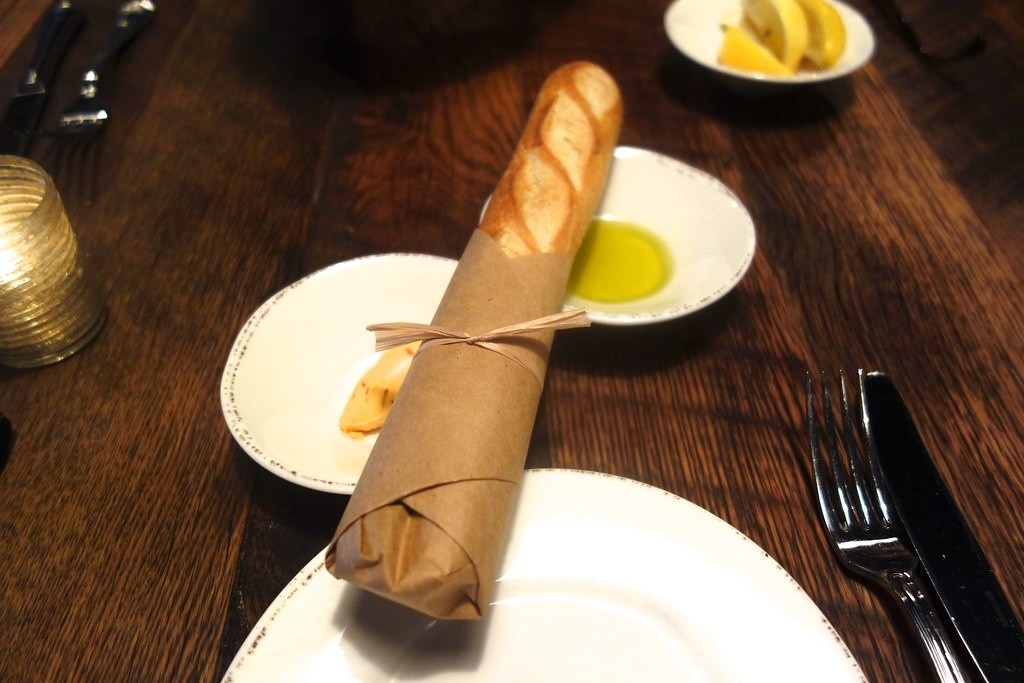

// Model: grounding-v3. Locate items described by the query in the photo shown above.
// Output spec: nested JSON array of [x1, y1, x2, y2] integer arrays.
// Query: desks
[[0, 0, 1024, 683]]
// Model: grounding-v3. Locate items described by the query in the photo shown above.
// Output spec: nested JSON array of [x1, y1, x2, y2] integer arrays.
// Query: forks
[[804, 368, 970, 683], [48, 0, 155, 212]]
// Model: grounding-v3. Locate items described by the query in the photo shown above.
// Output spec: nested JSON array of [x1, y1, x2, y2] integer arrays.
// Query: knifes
[[866, 372, 1024, 683], [0, 0, 84, 155]]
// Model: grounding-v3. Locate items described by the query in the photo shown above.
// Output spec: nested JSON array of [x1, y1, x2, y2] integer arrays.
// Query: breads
[[479, 58, 624, 260]]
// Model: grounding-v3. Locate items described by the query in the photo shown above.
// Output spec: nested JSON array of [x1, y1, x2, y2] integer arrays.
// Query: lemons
[[717, 0, 847, 78]]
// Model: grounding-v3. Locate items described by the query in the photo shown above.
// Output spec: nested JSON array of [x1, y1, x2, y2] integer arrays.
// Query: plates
[[663, 0, 875, 84], [220, 469, 868, 683], [221, 252, 459, 496], [479, 146, 757, 326]]
